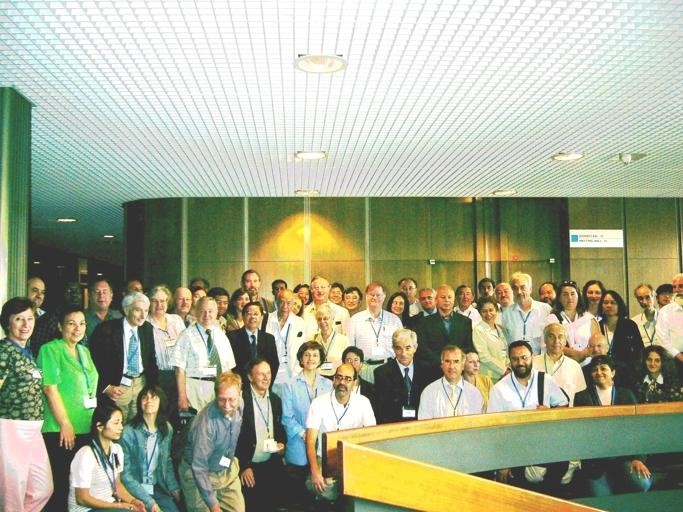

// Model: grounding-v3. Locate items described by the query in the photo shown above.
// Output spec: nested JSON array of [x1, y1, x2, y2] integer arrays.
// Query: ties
[[206, 329, 222, 376], [404, 368, 412, 392], [127, 330, 140, 377], [250, 334, 256, 359]]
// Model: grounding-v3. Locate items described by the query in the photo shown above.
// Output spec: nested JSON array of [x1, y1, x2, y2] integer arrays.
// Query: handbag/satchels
[[539, 371, 570, 408]]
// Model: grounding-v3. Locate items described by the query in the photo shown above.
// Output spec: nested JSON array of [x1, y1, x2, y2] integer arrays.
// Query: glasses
[[563, 280, 578, 291]]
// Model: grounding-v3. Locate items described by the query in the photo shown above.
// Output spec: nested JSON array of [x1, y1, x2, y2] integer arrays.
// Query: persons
[[0, 270, 683, 512]]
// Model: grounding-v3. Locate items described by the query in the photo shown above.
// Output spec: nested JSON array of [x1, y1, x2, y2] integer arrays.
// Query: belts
[[367, 358, 391, 364], [192, 377, 216, 381], [123, 371, 144, 379]]
